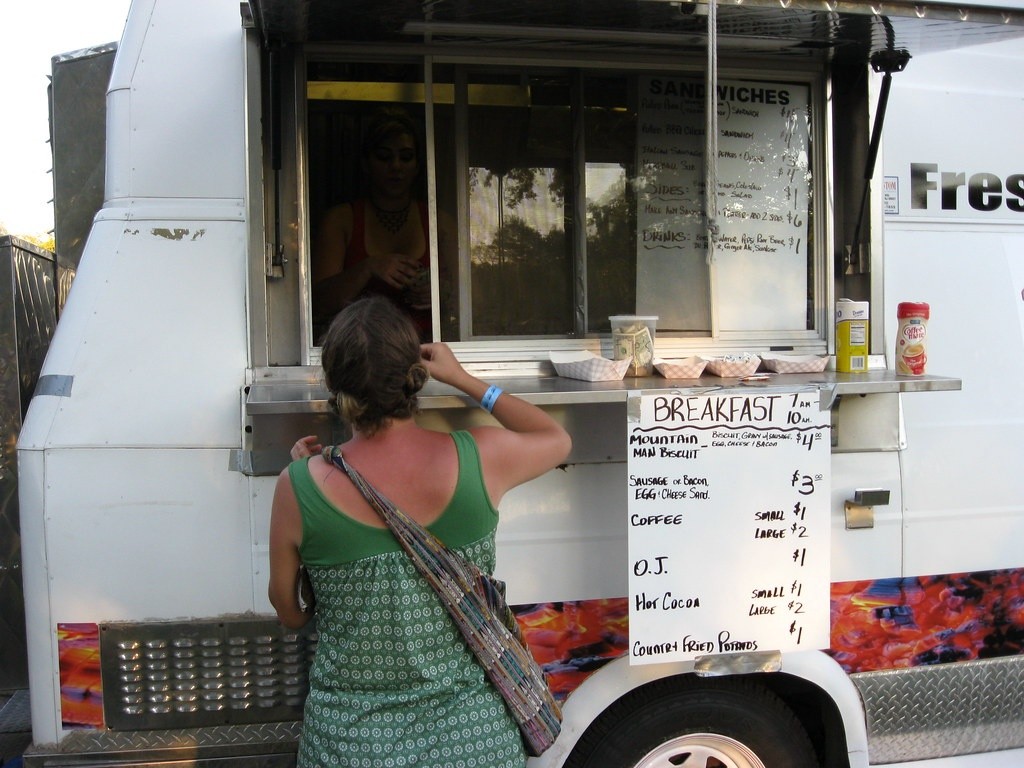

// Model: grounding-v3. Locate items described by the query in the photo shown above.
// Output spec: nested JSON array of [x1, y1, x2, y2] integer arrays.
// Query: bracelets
[[480, 385, 503, 413]]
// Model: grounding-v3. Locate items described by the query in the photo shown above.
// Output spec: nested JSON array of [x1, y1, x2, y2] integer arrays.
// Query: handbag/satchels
[[323, 445, 562, 757]]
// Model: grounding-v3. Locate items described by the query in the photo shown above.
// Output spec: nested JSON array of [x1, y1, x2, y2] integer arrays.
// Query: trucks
[[13, 3, 1023, 767]]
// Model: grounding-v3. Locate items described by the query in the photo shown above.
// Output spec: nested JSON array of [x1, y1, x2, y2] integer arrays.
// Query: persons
[[268, 298, 572, 768], [312, 106, 458, 346]]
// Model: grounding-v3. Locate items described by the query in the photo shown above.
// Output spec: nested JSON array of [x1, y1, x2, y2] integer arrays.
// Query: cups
[[609, 315, 659, 376], [896, 302, 930, 377]]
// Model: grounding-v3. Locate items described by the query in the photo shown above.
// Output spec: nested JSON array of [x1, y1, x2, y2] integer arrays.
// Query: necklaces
[[370, 199, 411, 234]]
